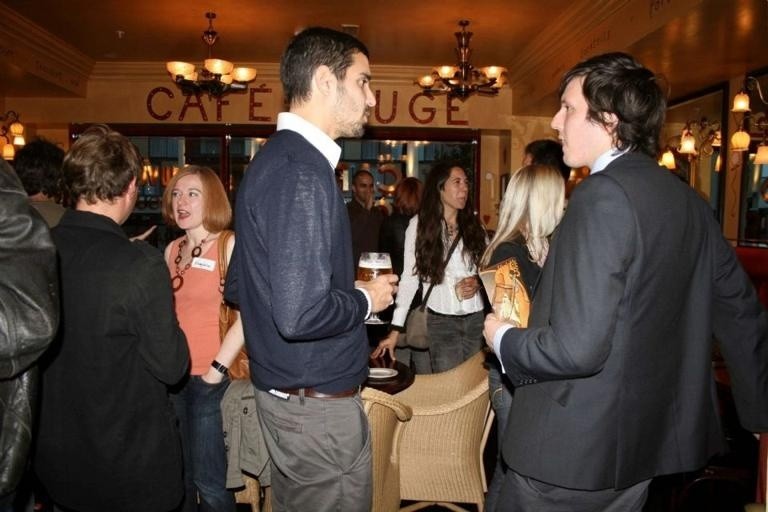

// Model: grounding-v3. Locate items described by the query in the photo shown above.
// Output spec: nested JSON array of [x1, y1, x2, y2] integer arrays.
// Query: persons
[[483, 51, 766, 511], [164, 163, 245, 511], [524, 139, 571, 183], [33, 126, 192, 510], [1, 154, 58, 510], [223, 26, 398, 510], [13, 140, 67, 228], [478, 165, 565, 510], [386, 176, 432, 374], [346, 169, 393, 368], [370, 156, 489, 373]]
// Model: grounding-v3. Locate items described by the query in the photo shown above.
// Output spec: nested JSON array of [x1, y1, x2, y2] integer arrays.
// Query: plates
[[368, 367, 399, 379]]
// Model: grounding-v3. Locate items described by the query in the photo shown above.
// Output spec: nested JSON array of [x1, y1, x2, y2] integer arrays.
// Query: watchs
[[211, 360, 228, 375]]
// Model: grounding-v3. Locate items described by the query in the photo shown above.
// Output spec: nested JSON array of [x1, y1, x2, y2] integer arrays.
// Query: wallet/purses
[[478, 255, 531, 329]]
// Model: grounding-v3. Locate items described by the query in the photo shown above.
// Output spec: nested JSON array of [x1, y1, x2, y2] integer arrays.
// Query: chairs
[[263, 387, 411, 512], [393, 351, 494, 511], [232, 471, 260, 511]]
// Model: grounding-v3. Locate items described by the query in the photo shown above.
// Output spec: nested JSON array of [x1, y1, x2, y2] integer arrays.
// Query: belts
[[426, 306, 476, 319], [269, 381, 361, 400]]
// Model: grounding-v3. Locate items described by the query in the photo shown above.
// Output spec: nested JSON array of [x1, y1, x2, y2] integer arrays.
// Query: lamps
[[416, 19, 508, 101], [658, 113, 726, 171], [165, 9, 257, 98], [0, 108, 27, 161], [735, 67, 767, 166]]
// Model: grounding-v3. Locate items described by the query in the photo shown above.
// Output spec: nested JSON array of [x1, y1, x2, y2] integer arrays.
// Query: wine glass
[[483, 283, 516, 353], [356, 253, 394, 325]]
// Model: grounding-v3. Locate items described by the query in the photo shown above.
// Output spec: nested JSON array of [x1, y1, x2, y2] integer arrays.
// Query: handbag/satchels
[[405, 225, 462, 351], [214, 227, 250, 382]]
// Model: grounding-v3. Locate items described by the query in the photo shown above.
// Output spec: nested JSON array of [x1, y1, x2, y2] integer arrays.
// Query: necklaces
[[171, 233, 209, 292]]
